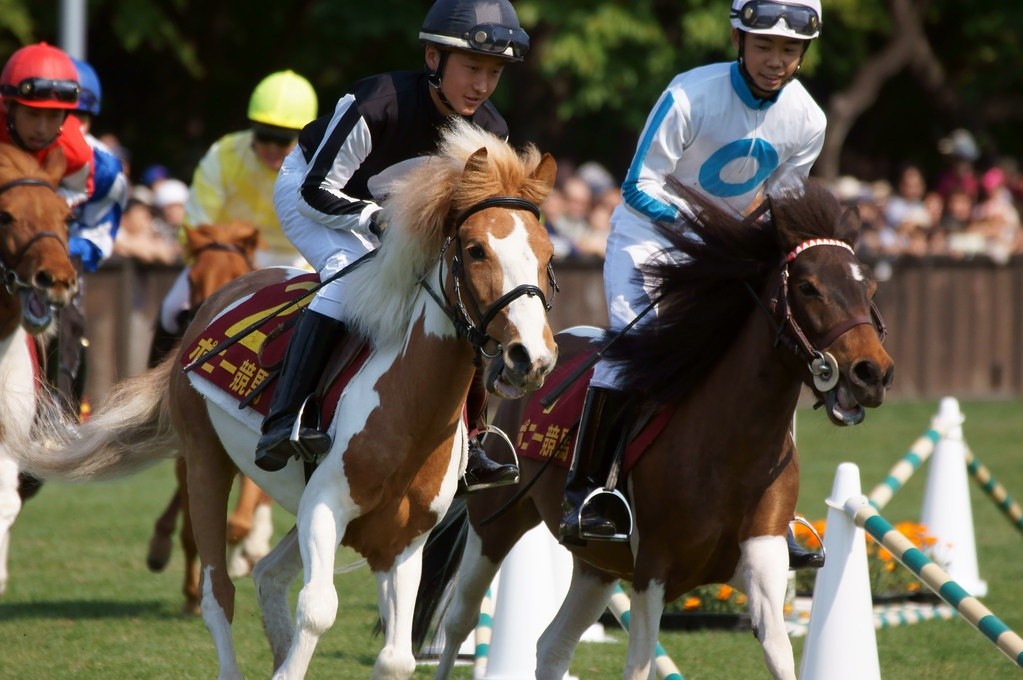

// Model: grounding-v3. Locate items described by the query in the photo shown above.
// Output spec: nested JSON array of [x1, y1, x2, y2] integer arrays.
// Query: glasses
[[741, 0, 819, 36], [251, 126, 294, 148], [466, 24, 530, 58], [18, 77, 80, 101], [80, 87, 100, 104]]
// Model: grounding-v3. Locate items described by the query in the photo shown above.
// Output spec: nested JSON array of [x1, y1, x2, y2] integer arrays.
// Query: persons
[[149, 71, 318, 372], [557, 0, 827, 569], [829, 131, 1023, 264], [542, 161, 621, 260], [100, 135, 190, 267], [255, 0, 531, 499], [0, 43, 127, 502]]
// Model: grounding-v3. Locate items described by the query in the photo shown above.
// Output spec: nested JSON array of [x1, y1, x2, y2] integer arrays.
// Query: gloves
[[369, 209, 388, 243]]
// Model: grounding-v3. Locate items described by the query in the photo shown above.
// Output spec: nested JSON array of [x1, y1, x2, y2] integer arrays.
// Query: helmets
[[70, 55, 101, 115], [248, 69, 318, 130], [729, 0, 822, 39], [0, 42, 81, 109], [418, 0, 525, 63]]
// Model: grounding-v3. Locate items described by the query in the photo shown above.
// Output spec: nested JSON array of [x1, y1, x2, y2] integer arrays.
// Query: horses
[[143, 119, 561, 680], [432, 201, 902, 680], [0, 145, 79, 593]]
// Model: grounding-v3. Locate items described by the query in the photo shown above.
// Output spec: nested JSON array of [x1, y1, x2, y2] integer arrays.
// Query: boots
[[149, 321, 176, 369], [254, 308, 348, 472], [558, 386, 631, 547], [454, 435, 519, 497], [787, 525, 824, 569]]
